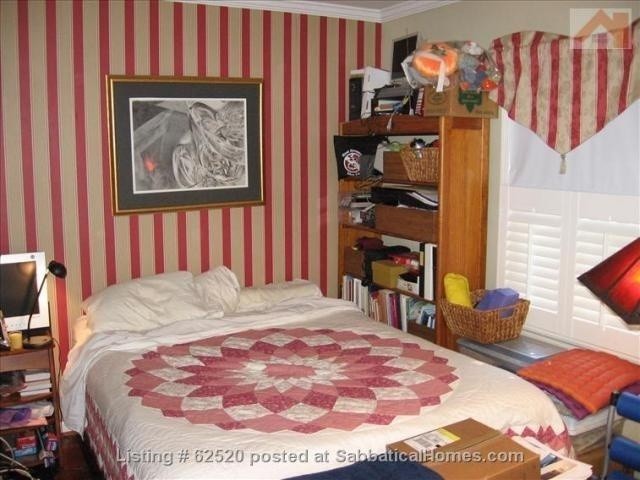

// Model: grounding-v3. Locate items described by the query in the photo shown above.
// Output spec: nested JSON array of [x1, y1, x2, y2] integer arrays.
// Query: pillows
[[80, 271, 207, 334]]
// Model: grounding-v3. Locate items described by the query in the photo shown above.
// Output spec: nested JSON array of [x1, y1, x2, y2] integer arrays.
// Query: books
[[416, 241, 437, 300], [404, 191, 439, 210], [342, 275, 411, 334], [14, 368, 53, 398]]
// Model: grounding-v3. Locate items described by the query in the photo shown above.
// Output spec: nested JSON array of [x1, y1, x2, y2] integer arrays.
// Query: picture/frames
[[104, 73, 266, 217]]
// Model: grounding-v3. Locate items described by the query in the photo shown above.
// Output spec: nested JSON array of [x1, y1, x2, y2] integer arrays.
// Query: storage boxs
[[424, 78, 498, 119], [384, 417, 541, 480]]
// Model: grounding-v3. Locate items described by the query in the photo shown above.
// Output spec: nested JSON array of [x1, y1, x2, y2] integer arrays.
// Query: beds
[[58, 264, 576, 480]]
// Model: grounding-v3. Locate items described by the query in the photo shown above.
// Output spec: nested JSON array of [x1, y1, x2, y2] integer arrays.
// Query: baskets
[[441, 289, 530, 344], [400, 148, 440, 183]]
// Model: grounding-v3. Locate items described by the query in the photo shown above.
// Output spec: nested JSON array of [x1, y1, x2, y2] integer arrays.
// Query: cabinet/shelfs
[[338, 116, 490, 344], [1, 341, 63, 473]]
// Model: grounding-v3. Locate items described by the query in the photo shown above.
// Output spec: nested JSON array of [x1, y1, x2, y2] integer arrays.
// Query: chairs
[[600, 391, 639, 479]]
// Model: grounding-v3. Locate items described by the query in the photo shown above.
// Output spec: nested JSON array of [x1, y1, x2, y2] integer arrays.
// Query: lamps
[[22, 260, 67, 350]]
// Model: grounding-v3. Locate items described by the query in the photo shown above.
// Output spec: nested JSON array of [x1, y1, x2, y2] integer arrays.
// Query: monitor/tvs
[[0, 250, 52, 333]]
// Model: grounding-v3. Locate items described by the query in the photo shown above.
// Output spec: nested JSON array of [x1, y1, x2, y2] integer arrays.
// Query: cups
[[8, 331, 23, 352]]
